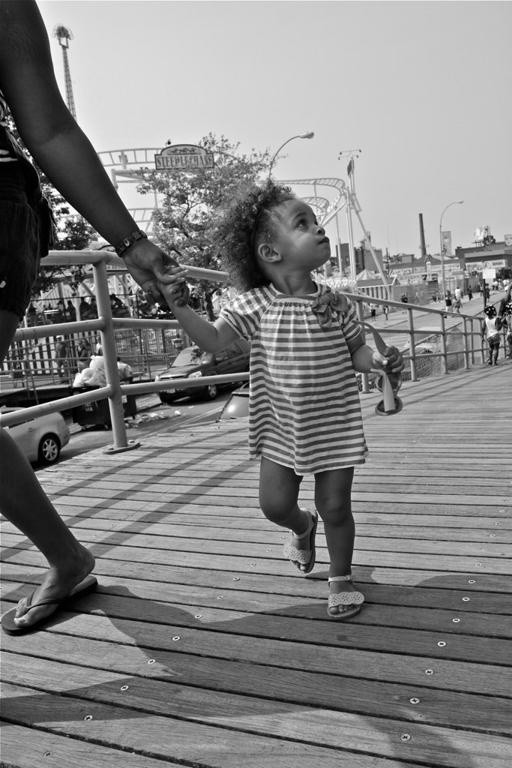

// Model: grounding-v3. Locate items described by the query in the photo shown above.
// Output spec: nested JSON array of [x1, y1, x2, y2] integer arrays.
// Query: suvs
[[151, 343, 251, 405]]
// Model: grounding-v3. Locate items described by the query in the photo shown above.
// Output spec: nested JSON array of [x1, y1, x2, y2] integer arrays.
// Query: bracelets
[[115, 230, 146, 255]]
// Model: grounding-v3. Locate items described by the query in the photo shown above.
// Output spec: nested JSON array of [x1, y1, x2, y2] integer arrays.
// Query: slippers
[[0, 575, 98, 636]]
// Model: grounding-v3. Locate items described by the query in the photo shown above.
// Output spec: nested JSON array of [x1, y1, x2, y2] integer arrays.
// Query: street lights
[[439, 199, 466, 310], [336, 148, 364, 193], [50, 21, 81, 126], [264, 132, 320, 186]]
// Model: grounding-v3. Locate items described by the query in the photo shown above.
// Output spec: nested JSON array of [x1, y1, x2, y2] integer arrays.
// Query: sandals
[[327, 575, 365, 617], [287, 507, 318, 574]]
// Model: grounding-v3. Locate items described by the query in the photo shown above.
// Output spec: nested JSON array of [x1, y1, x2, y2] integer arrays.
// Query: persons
[[162, 184, 404, 619], [370, 275, 512, 366], [1, 0, 189, 636], [24, 294, 128, 324], [55, 335, 132, 387]]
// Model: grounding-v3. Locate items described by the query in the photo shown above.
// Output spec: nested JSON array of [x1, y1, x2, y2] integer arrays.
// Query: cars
[[1, 405, 72, 468], [357, 324, 466, 389], [212, 383, 252, 423]]
